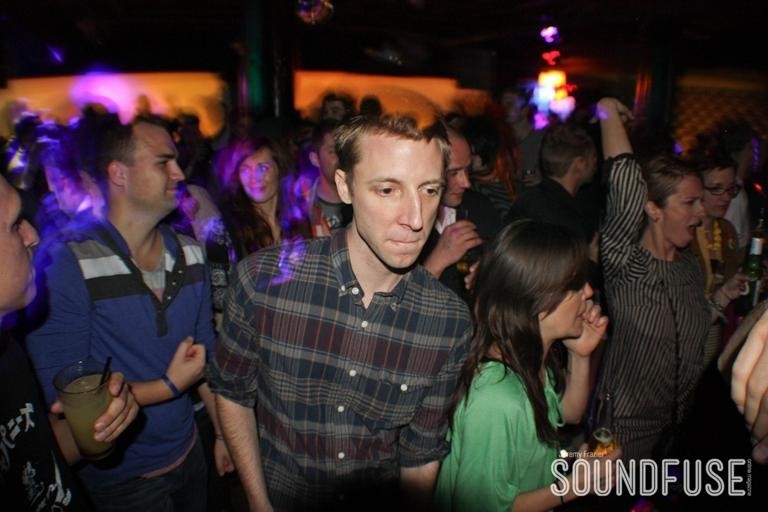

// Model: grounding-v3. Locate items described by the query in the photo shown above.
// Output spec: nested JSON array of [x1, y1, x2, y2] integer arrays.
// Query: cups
[[455, 246, 482, 290], [49, 361, 117, 465]]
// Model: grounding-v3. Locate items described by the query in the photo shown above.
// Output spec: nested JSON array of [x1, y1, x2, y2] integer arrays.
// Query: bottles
[[733, 230, 764, 316], [586, 392, 615, 458]]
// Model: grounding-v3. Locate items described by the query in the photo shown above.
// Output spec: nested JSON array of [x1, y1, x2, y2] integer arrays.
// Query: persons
[[1, 82, 767, 509]]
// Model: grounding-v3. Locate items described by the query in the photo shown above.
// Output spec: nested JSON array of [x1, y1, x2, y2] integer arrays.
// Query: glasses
[[704, 185, 740, 197]]
